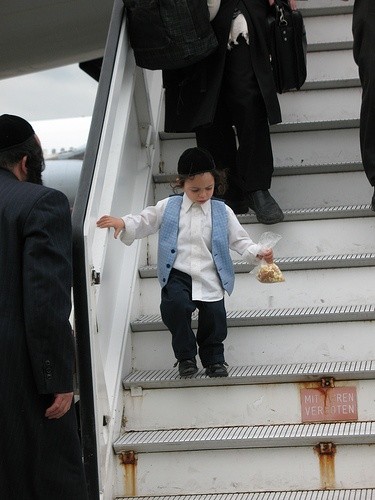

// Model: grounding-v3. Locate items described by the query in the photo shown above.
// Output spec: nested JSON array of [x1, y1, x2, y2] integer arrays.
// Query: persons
[[0, 113, 87, 500], [96, 146, 274, 379], [157, 0, 307, 225]]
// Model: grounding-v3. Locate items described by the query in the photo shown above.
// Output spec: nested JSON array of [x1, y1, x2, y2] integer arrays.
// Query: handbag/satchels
[[270, 0, 306, 94]]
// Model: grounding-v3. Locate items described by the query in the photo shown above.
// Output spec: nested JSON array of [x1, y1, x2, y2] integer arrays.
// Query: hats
[[178, 147, 215, 182], [0, 114, 34, 151]]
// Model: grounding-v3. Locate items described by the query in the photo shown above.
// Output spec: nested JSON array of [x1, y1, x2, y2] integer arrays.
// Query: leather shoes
[[248, 189, 284, 225], [179, 357, 198, 376], [205, 364, 228, 377]]
